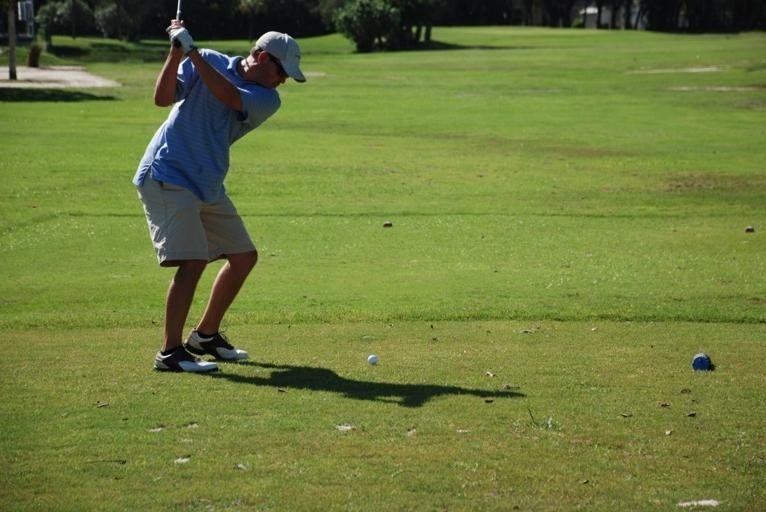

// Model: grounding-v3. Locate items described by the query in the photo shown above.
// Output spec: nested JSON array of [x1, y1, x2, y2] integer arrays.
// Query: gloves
[[168, 27, 197, 58]]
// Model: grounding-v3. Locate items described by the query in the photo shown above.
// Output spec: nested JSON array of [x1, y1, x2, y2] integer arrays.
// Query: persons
[[130, 19, 309, 376]]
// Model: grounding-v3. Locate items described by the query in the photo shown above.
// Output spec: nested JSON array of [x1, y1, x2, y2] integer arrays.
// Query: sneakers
[[184, 328, 249, 360], [153, 345, 218, 373]]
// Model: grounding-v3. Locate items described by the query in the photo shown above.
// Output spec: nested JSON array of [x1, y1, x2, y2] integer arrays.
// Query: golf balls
[[367, 354, 377, 367]]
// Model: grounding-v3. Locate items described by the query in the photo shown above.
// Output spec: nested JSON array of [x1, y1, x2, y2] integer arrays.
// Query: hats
[[256, 30, 306, 83]]
